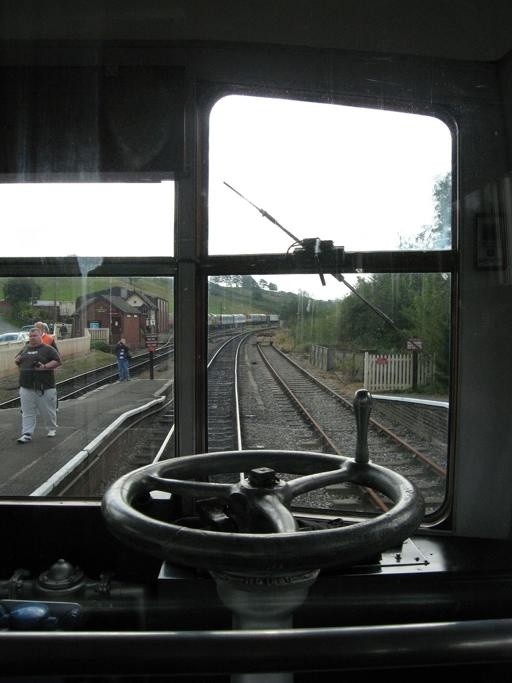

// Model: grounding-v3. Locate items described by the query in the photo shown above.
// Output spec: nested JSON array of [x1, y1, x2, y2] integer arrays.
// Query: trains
[[208, 314, 280, 327]]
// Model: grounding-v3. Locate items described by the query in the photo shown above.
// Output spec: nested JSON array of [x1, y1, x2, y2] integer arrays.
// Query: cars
[[0, 325, 36, 344]]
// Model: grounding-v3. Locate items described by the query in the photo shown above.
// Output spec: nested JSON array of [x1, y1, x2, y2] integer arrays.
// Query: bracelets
[[44, 364, 47, 368]]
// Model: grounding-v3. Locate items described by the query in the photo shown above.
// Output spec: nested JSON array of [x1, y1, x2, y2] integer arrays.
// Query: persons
[[114, 336, 132, 381], [20, 320, 61, 414], [14, 327, 63, 443], [59, 322, 68, 339]]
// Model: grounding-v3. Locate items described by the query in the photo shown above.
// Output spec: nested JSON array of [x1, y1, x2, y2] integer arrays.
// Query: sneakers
[[48, 429, 56, 437], [17, 435, 32, 443]]
